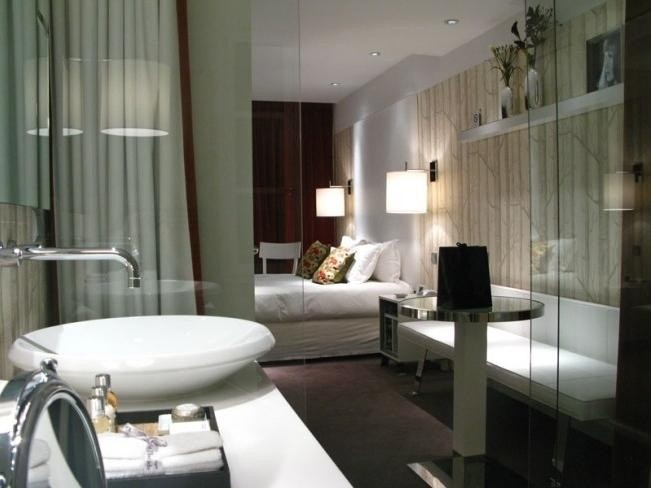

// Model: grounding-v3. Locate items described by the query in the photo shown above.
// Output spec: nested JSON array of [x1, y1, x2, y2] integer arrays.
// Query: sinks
[[7, 314, 277, 402]]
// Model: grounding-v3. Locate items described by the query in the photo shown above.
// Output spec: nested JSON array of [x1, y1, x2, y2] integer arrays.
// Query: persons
[[599, 36, 621, 90]]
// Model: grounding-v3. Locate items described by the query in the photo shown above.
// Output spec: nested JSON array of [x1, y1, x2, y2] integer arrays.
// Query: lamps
[[384, 161, 438, 215], [315, 178, 352, 218], [602, 163, 645, 212], [98, 58, 173, 142], [22, 57, 83, 139]]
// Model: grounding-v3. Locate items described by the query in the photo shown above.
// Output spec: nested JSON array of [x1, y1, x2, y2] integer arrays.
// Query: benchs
[[399, 282, 620, 474]]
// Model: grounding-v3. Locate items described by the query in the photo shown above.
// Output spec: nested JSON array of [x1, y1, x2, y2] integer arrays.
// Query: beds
[[254, 274, 412, 364]]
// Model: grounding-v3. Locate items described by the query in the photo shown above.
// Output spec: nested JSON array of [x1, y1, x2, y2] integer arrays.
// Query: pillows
[[297, 235, 404, 285]]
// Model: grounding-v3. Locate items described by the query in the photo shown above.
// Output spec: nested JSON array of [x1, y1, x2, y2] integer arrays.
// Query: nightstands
[[376, 292, 450, 372]]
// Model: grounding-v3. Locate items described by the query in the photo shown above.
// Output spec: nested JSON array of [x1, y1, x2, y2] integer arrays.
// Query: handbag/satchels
[[437, 242, 491, 312]]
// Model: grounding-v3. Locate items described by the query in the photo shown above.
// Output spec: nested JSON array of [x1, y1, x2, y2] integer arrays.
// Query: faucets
[[0, 238, 141, 290]]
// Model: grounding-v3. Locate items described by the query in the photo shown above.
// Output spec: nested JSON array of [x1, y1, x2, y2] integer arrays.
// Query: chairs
[[259, 242, 302, 274]]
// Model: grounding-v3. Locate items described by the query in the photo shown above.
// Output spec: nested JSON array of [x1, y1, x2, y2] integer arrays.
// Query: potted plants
[[511, 3, 564, 110], [487, 39, 522, 120]]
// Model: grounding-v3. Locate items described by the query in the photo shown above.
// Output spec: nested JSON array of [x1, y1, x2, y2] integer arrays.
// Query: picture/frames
[[585, 24, 622, 93]]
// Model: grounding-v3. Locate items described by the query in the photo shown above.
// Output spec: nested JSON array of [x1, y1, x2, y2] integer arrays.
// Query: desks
[[399, 294, 544, 458]]
